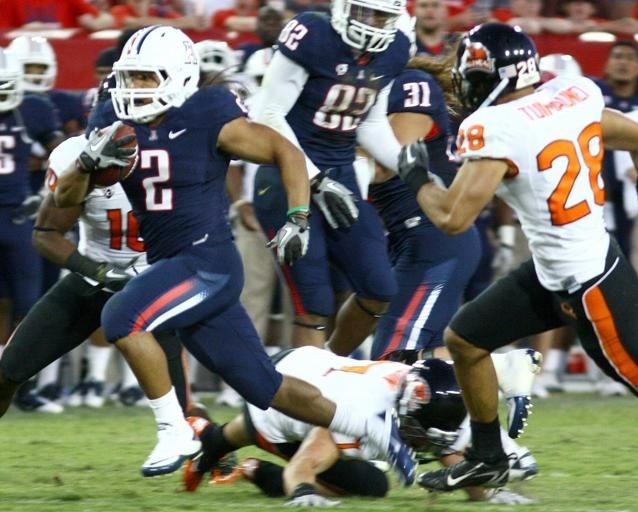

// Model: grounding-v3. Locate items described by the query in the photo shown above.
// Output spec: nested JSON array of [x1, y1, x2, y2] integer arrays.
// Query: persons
[[3, 1, 637, 510]]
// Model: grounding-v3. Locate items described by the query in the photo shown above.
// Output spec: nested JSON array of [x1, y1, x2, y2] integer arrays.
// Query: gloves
[[83, 253, 140, 296], [394, 140, 431, 198], [309, 172, 360, 232], [480, 486, 535, 506], [8, 194, 44, 225], [282, 481, 341, 509], [264, 216, 310, 269], [77, 119, 138, 172]]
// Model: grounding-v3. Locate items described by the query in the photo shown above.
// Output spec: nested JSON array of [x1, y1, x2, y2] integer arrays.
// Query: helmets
[[5, 33, 57, 93], [105, 24, 201, 126], [330, 1, 404, 53], [196, 39, 239, 83], [448, 21, 543, 114], [0, 47, 26, 113], [393, 358, 468, 451]]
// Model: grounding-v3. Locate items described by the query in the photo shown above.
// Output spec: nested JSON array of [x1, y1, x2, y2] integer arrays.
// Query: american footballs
[[90, 126, 139, 187]]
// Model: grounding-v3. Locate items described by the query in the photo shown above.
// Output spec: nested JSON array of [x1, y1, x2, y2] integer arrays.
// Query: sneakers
[[507, 443, 540, 483], [356, 399, 420, 489], [12, 391, 64, 415], [139, 422, 205, 479], [35, 378, 69, 406], [498, 347, 544, 439], [65, 376, 93, 408], [414, 446, 518, 491], [180, 415, 218, 493], [208, 451, 243, 486], [214, 385, 246, 411], [117, 384, 153, 407], [239, 457, 283, 499], [82, 376, 108, 408]]
